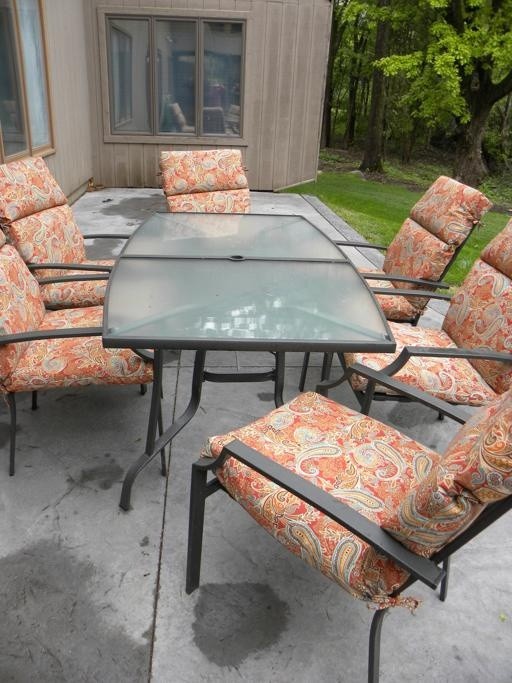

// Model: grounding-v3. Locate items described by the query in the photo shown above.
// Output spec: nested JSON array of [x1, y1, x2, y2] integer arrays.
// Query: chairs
[[0, 228, 167, 476], [0, 156, 132, 310], [322, 218, 512, 419], [185, 384, 512, 682], [170, 101, 240, 136], [299, 175, 493, 392], [158, 148, 251, 213]]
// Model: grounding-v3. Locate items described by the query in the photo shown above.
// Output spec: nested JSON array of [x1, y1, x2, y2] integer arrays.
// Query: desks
[[102, 211, 396, 511]]
